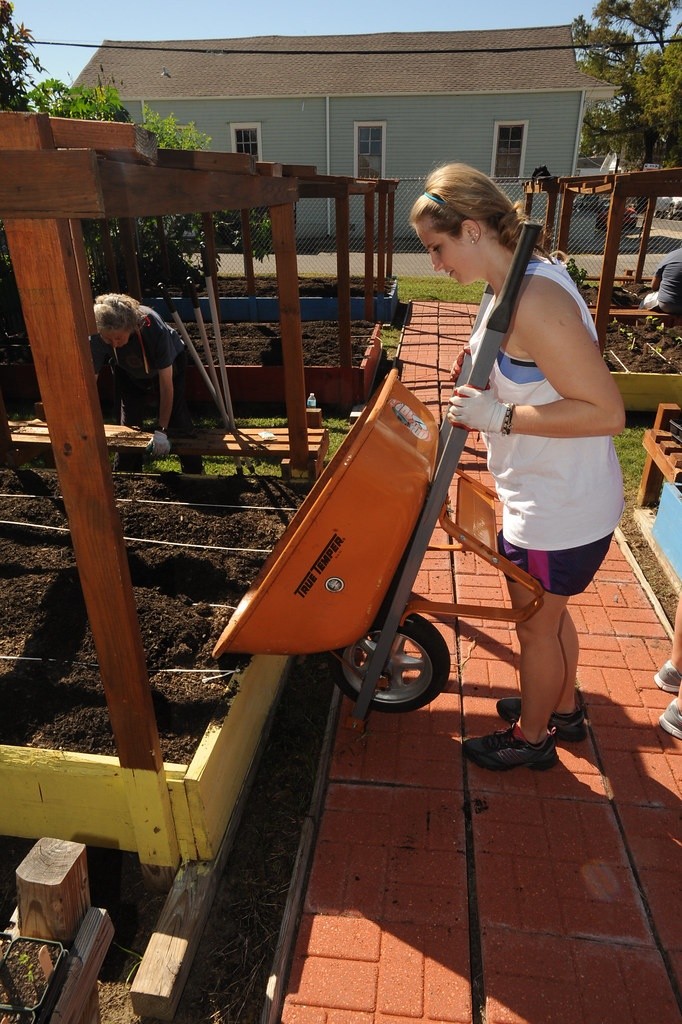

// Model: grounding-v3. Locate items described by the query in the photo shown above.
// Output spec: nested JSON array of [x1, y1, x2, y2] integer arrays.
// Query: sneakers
[[654, 659, 682, 692], [496, 687, 587, 742], [659, 695, 682, 740], [462, 722, 557, 771]]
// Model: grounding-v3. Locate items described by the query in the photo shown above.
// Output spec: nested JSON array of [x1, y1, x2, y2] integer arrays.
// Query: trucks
[[655, 194, 682, 220]]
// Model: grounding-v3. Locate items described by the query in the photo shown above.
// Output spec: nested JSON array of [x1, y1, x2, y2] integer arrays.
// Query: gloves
[[447, 379, 507, 434], [450, 346, 471, 379], [146, 430, 170, 456]]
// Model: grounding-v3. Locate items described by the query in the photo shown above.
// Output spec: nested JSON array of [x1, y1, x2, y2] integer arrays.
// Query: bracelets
[[156, 426, 167, 433], [502, 403, 515, 436]]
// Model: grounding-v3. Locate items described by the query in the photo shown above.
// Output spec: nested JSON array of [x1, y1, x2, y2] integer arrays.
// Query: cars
[[596, 202, 637, 230], [572, 192, 603, 212]]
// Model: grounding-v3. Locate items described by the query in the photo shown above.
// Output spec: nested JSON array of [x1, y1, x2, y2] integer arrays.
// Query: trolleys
[[210, 212, 548, 714]]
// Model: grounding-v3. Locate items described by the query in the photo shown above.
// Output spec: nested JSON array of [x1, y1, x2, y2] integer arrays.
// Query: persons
[[411, 163, 626, 771], [654, 592, 682, 738], [89, 294, 203, 474], [651, 247, 682, 313]]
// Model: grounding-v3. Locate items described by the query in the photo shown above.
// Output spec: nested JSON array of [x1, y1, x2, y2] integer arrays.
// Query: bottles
[[307, 393, 316, 408]]
